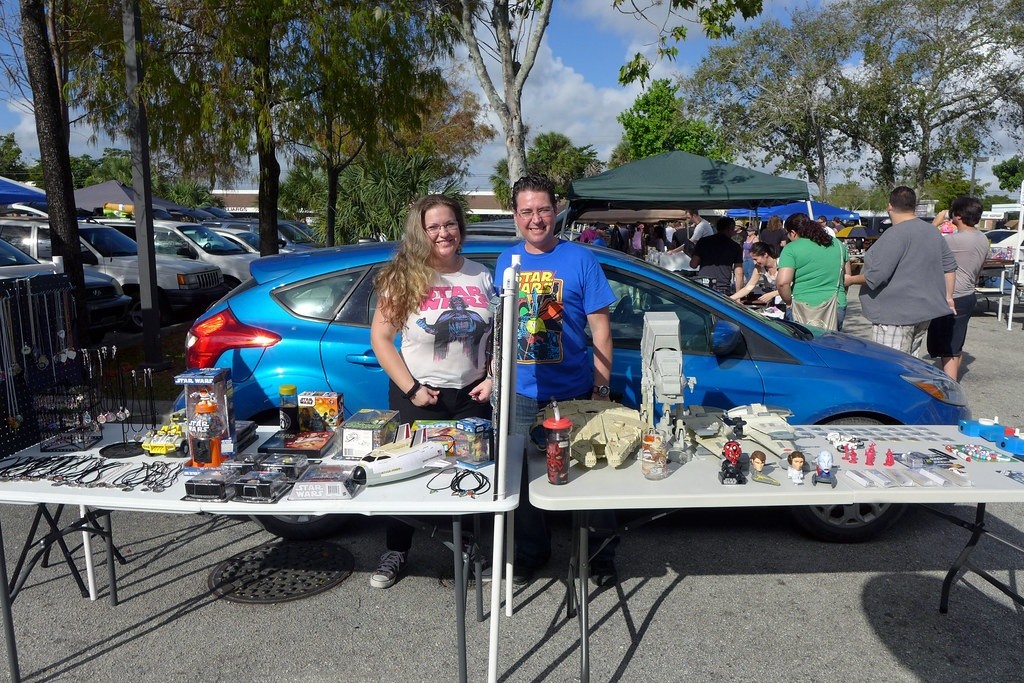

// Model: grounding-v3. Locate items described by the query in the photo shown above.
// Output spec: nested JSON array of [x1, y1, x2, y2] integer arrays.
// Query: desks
[[526, 423, 1023, 683], [0, 424, 524, 683]]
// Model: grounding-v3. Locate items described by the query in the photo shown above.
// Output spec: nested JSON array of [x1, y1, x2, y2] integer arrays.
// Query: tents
[[0, 180, 47, 209], [73, 180, 183, 211], [561, 151, 814, 238], [727, 200, 861, 234]]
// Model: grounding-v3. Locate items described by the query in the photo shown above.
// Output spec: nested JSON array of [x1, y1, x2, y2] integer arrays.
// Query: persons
[[576, 206, 791, 313], [844, 187, 958, 357], [926, 199, 990, 381], [370, 193, 494, 588], [775, 215, 851, 331], [750, 450, 767, 472], [788, 451, 806, 483], [818, 451, 833, 478], [723, 441, 744, 482], [495, 176, 618, 587], [818, 216, 871, 272]]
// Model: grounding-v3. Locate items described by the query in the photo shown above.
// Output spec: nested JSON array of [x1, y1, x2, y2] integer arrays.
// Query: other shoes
[[513, 558, 536, 584], [590, 560, 617, 588]]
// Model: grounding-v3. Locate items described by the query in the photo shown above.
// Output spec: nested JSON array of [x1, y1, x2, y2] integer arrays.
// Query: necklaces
[[0, 277, 182, 491]]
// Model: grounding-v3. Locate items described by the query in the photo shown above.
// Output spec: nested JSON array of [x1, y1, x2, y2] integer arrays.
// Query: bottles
[[279, 386, 299, 437]]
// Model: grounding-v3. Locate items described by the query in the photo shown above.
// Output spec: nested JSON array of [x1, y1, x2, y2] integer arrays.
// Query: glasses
[[426, 221, 458, 232], [517, 206, 554, 219]]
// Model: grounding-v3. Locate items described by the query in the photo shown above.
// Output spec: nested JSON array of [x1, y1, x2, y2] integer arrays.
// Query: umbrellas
[[836, 224, 880, 240]]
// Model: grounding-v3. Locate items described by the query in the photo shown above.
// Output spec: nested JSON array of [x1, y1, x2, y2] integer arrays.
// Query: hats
[[746, 227, 756, 231], [735, 220, 743, 226]]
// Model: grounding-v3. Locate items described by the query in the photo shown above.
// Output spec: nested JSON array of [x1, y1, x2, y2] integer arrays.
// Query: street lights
[[970, 157, 989, 198]]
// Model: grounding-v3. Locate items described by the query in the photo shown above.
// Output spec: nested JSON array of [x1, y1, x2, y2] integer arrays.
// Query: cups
[[542, 416, 573, 486], [641, 428, 668, 480]]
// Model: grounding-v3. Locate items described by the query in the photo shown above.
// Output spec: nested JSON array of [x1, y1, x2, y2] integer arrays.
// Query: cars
[[77, 217, 261, 292], [102, 200, 327, 256], [167, 236, 975, 545], [0, 216, 230, 327], [462, 209, 565, 236], [0, 238, 135, 374], [851, 204, 1024, 305]]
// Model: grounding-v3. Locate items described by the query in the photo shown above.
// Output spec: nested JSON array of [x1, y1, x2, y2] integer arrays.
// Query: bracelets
[[401, 380, 420, 399]]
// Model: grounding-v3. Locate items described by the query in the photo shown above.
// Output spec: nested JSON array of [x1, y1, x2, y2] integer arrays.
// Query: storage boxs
[[297, 392, 343, 432], [456, 416, 492, 462], [336, 409, 400, 458]]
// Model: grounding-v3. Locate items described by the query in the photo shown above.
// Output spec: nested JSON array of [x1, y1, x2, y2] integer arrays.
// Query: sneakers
[[465, 544, 492, 582], [370, 550, 407, 588]]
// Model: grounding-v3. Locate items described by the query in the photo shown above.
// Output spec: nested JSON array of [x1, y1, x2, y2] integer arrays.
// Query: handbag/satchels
[[792, 293, 837, 331]]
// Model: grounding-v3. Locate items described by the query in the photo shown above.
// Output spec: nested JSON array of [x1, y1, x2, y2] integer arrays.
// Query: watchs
[[593, 385, 611, 396]]
